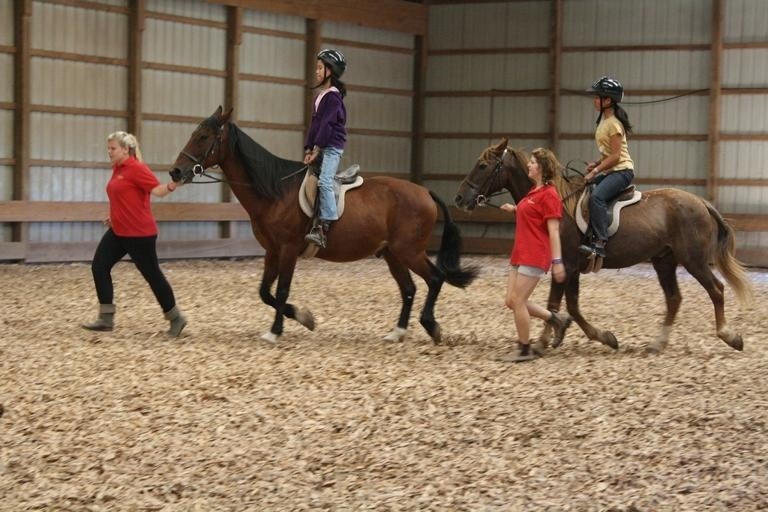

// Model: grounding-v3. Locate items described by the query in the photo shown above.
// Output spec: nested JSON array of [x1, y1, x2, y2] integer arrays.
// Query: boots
[[83, 304, 116, 331], [545, 312, 571, 348], [305, 219, 332, 249], [165, 305, 187, 337], [502, 341, 534, 361]]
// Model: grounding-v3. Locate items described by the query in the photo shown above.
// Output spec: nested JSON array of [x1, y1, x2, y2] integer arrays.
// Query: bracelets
[[552, 258, 562, 264], [593, 161, 598, 165], [167, 182, 176, 192], [304, 145, 320, 154], [594, 168, 599, 173]]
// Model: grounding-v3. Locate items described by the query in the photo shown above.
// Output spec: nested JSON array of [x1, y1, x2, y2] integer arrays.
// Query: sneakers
[[578, 242, 606, 259]]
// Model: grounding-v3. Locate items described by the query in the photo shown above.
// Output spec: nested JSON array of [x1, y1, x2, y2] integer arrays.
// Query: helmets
[[317, 49, 346, 78], [587, 77, 623, 101]]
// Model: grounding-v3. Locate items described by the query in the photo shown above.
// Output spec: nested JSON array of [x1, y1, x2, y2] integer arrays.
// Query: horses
[[169, 105, 483, 345], [454, 137, 753, 357]]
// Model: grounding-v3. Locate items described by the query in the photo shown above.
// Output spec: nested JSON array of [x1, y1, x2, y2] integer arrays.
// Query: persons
[[577, 76, 635, 258], [80, 131, 187, 337], [303, 48, 347, 249], [496, 147, 571, 362]]
